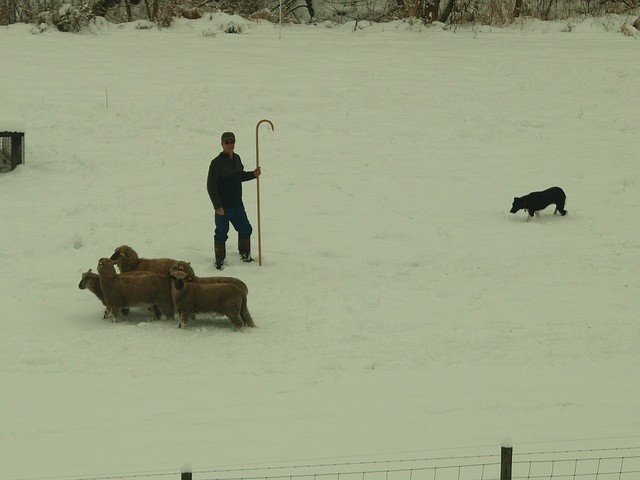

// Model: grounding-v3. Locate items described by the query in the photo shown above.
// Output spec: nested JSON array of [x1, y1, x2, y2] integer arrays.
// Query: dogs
[[511, 187, 567, 223]]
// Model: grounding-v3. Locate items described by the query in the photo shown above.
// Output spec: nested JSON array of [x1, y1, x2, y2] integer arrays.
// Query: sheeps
[[97, 259, 175, 323], [168, 270, 247, 332], [110, 246, 179, 279], [79, 268, 161, 320], [168, 259, 255, 327]]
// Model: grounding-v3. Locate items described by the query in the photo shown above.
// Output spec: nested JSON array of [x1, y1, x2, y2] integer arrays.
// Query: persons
[[207, 132, 261, 270]]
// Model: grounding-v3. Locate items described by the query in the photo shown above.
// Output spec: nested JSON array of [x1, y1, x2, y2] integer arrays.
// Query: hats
[[221, 131, 235, 145]]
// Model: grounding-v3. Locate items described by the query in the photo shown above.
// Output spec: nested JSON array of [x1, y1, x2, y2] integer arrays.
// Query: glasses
[[225, 140, 235, 144]]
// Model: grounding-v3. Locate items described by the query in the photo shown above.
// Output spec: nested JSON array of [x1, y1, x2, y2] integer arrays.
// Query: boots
[[238, 238, 254, 262], [215, 244, 226, 270]]
